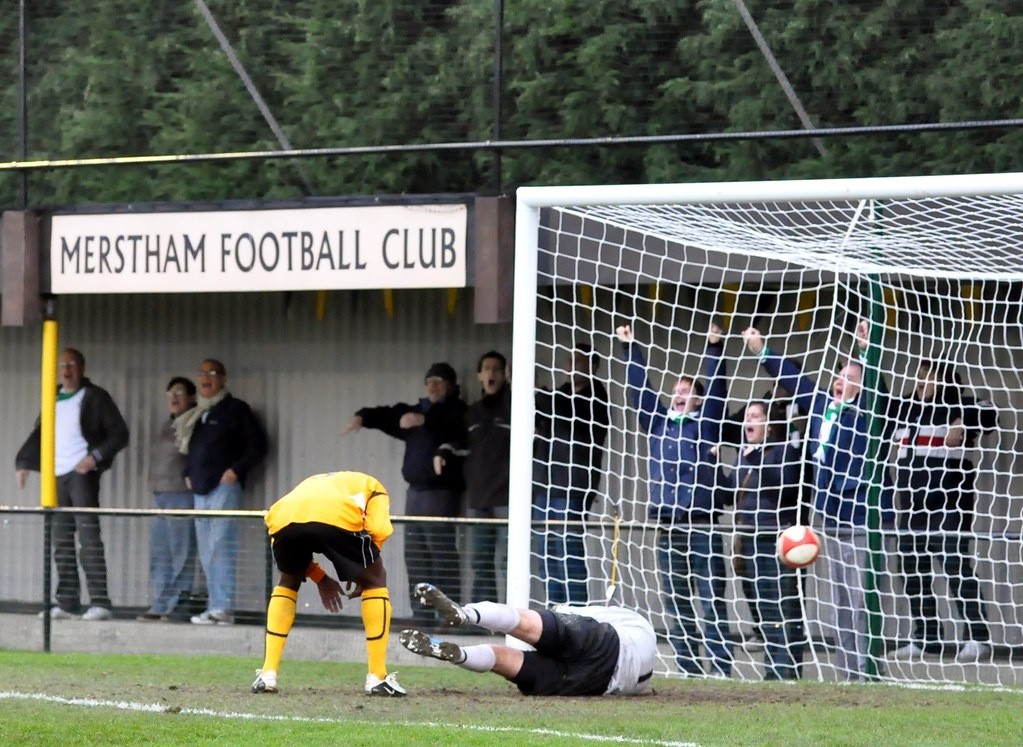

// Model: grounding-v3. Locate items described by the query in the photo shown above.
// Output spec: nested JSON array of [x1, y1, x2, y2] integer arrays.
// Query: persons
[[252, 471, 407, 697], [615, 321, 999, 683], [340, 343, 612, 627], [136, 358, 270, 626], [399, 582, 657, 697], [15, 348, 129, 621]]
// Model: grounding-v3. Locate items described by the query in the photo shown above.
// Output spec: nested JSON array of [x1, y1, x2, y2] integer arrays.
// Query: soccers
[[777, 526, 821, 568]]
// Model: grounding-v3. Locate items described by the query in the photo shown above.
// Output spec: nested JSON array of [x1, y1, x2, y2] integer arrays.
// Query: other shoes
[[889, 644, 942, 659], [141, 609, 185, 622], [959, 640, 992, 661], [192, 609, 233, 626], [82, 605, 111, 620], [38, 606, 77, 621]]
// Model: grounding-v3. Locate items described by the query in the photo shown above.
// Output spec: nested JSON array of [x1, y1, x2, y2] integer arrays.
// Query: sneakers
[[251, 671, 279, 693], [365, 670, 409, 697], [397, 629, 464, 665], [414, 583, 468, 629]]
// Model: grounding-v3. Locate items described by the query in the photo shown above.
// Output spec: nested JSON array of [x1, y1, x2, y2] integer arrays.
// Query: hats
[[424, 363, 457, 387]]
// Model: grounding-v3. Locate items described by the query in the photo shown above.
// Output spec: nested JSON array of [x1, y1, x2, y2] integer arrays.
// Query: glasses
[[58, 359, 77, 367], [197, 370, 219, 377], [165, 390, 185, 398]]
